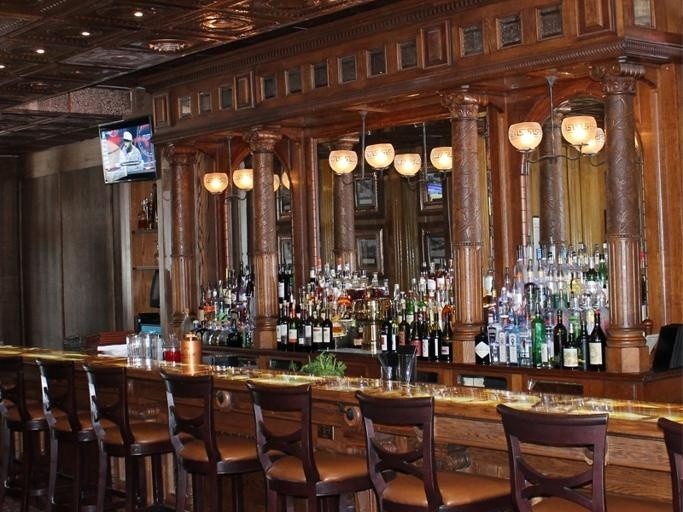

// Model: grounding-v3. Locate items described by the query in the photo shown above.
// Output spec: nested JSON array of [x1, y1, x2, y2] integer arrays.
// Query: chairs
[[1, 356, 89, 507], [243, 378, 376, 512], [355, 387, 513, 512], [157, 368, 267, 512], [40, 355, 162, 505], [494, 404, 673, 512], [83, 364, 192, 512]]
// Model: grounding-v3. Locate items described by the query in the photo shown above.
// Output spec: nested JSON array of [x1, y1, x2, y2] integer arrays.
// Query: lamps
[[393, 121, 453, 190], [508, 76, 597, 168], [202, 135, 254, 200], [576, 125, 642, 167], [328, 110, 394, 184]]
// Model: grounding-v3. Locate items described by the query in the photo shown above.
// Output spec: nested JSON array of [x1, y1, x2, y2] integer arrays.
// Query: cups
[[124, 331, 162, 371], [380, 365, 397, 391], [398, 352, 419, 399]]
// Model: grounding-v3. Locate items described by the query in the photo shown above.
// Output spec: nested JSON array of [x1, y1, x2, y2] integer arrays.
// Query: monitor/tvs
[[97, 113, 161, 185]]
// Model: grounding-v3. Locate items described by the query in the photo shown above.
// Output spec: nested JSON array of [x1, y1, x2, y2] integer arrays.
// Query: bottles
[[275, 258, 455, 362], [476, 235, 611, 371], [181, 261, 256, 349]]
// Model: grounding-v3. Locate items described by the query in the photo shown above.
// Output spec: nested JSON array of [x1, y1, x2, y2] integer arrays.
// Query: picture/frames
[[353, 172, 381, 217], [275, 186, 292, 223], [420, 176, 446, 214], [276, 234, 293, 269], [353, 228, 384, 276], [420, 223, 447, 270]]
